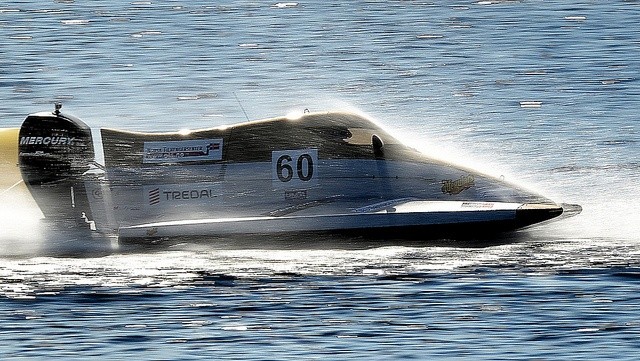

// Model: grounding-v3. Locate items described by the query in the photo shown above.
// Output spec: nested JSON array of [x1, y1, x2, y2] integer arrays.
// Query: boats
[[18, 92, 583, 252]]
